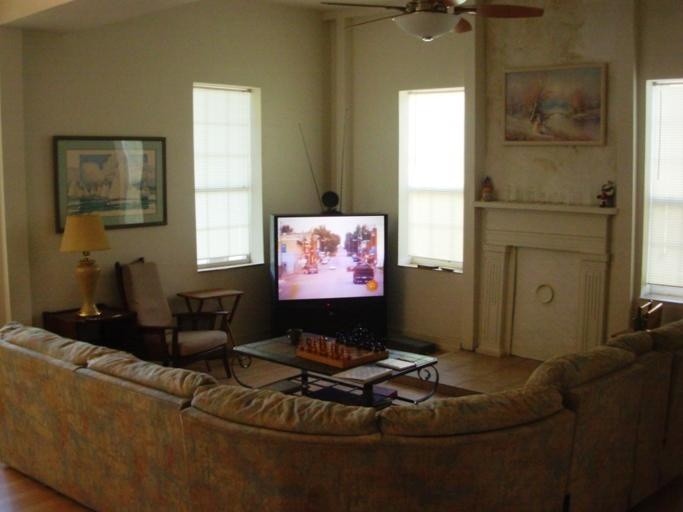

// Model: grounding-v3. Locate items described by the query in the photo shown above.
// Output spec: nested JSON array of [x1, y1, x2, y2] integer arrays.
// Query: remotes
[[440, 268, 453, 272]]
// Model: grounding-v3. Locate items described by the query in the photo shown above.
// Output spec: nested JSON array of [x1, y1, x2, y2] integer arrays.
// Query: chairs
[[116, 255, 231, 379]]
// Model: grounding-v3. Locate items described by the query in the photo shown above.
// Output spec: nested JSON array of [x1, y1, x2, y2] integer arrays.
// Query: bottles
[[480, 176, 492, 201]]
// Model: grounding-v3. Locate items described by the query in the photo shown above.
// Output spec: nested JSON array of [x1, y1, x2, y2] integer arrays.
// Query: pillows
[[122, 261, 172, 328]]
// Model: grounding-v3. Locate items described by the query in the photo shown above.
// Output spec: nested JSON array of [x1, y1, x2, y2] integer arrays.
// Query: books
[[331, 365, 393, 384], [375, 357, 415, 371]]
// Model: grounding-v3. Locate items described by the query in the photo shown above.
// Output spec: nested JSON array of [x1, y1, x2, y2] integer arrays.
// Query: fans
[[324, 2, 543, 33]]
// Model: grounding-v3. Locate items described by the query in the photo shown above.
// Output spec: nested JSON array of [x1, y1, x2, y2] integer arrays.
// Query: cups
[[286, 326, 302, 344]]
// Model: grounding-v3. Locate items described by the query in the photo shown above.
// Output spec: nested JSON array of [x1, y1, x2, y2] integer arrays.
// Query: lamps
[[59, 214, 112, 317], [390, 12, 460, 40]]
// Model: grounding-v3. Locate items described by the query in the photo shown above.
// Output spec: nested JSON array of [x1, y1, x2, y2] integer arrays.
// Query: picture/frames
[[502, 63, 606, 146], [53, 136, 166, 234]]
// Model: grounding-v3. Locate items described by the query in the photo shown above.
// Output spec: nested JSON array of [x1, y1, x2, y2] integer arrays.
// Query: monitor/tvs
[[269, 213, 388, 305]]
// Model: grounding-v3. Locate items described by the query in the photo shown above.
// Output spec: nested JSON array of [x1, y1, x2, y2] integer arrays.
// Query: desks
[[176, 288, 251, 369]]
